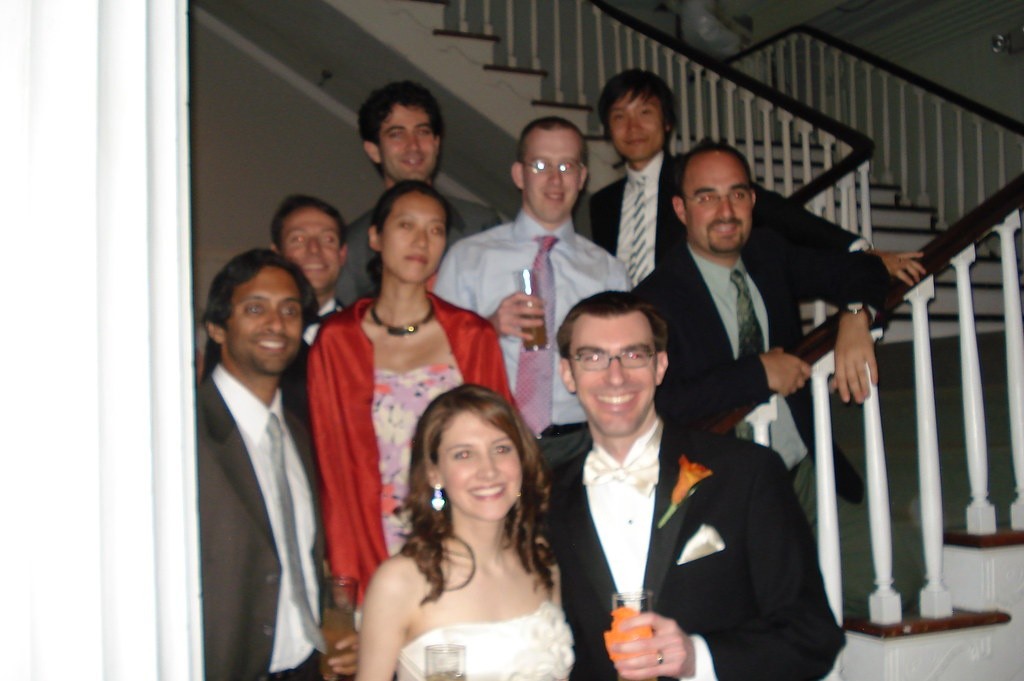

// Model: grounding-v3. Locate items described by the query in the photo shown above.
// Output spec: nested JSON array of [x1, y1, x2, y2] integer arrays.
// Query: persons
[[433, 116, 632, 435], [193, 247, 360, 681], [536, 288, 847, 681], [355, 382, 574, 681], [336, 80, 504, 304], [589, 69, 926, 289], [632, 141, 878, 436], [307, 178, 516, 606], [270, 195, 349, 418]]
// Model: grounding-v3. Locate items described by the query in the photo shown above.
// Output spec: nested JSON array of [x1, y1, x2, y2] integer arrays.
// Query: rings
[[657, 652, 663, 664], [899, 257, 903, 263]]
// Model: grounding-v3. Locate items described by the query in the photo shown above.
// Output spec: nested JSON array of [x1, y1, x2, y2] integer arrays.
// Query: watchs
[[845, 302, 863, 315]]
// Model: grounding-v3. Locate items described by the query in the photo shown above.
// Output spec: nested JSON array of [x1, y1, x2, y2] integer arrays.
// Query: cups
[[513, 268, 550, 352], [613, 589, 653, 616], [320, 576, 356, 675], [425, 644, 465, 681]]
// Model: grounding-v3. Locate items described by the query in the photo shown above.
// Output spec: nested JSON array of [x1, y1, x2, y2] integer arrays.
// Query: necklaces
[[370, 297, 434, 336]]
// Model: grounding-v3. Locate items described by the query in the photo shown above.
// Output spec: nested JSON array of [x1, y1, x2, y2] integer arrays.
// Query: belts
[[265, 649, 317, 681], [541, 423, 588, 437]]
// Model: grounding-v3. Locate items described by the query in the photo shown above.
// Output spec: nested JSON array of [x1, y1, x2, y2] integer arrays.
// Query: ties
[[627, 175, 649, 289], [513, 235, 558, 435], [267, 411, 328, 655], [730, 269, 764, 441]]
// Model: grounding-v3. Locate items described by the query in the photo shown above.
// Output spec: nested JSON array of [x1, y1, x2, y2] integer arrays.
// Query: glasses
[[682, 189, 750, 207], [568, 348, 655, 371], [522, 160, 580, 175]]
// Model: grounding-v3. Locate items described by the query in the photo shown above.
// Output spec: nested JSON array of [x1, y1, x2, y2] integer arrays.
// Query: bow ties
[[308, 311, 335, 325], [579, 418, 661, 497]]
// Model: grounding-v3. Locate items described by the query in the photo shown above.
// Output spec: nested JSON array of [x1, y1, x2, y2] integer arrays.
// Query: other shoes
[[829, 446, 862, 504]]
